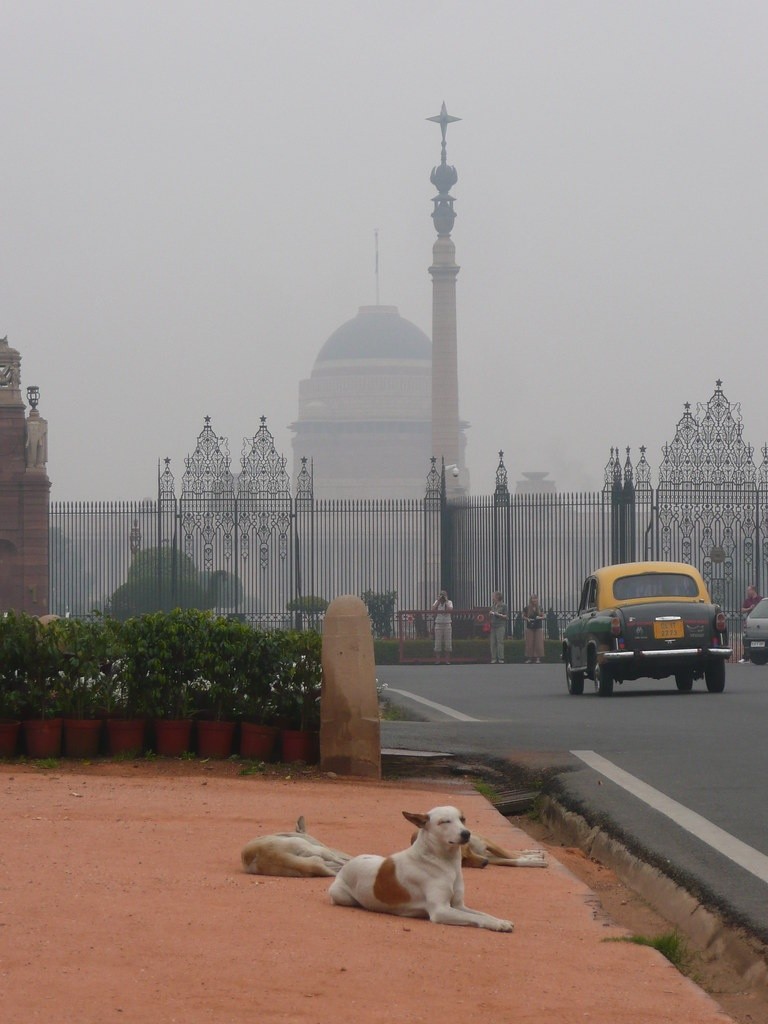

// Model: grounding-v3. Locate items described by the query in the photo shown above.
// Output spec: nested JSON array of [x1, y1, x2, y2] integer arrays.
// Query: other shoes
[[525, 658, 532, 664], [491, 659, 497, 664], [738, 658, 753, 664], [498, 659, 504, 663], [535, 658, 541, 664]]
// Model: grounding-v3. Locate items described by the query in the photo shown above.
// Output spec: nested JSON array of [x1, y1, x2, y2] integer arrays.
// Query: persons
[[739, 585, 763, 664], [488, 592, 508, 663], [432, 591, 453, 664], [522, 594, 546, 663]]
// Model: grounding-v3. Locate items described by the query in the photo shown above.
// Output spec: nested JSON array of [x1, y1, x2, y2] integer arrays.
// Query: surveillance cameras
[[452, 467, 460, 477]]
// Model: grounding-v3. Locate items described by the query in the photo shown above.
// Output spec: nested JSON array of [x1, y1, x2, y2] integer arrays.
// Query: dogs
[[242, 804, 549, 932]]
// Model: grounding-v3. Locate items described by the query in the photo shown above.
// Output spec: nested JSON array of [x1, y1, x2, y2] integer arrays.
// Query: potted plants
[[0, 607, 323, 767]]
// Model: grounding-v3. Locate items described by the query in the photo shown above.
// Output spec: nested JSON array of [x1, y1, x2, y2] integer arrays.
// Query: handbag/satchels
[[483, 616, 491, 632], [527, 616, 542, 628]]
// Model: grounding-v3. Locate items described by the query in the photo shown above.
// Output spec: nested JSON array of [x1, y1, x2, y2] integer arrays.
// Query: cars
[[561, 561, 734, 697], [741, 598, 768, 667]]
